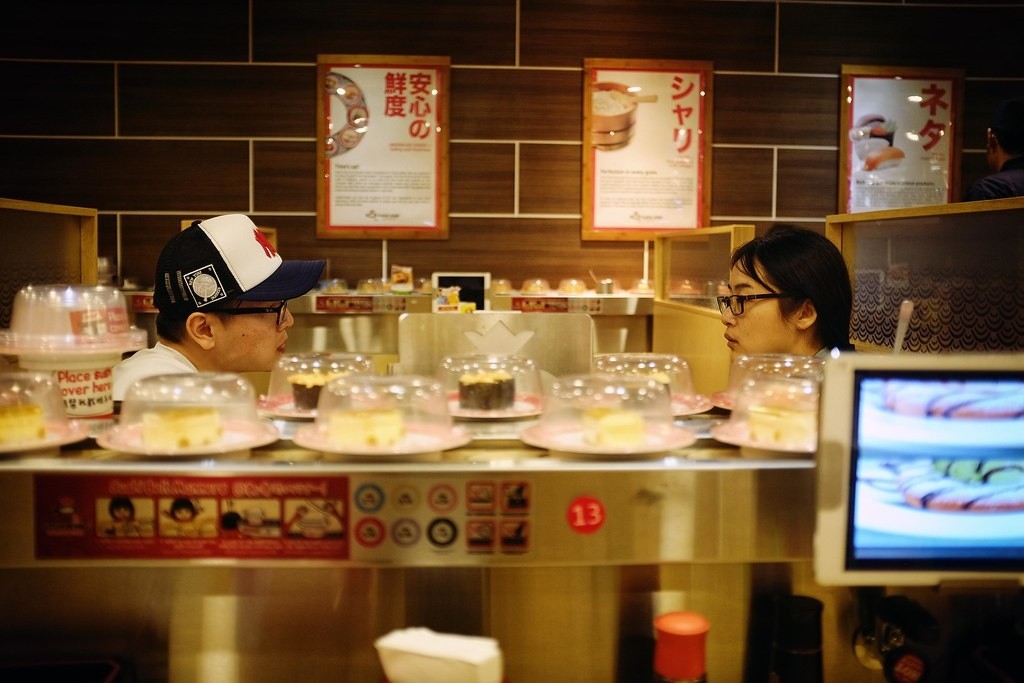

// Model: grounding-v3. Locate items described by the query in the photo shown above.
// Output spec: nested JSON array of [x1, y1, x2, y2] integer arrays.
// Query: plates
[[293, 422, 472, 459], [670, 394, 715, 419], [849, 126, 871, 143], [446, 390, 541, 420], [1, 428, 90, 459], [96, 421, 279, 461], [522, 424, 700, 459], [258, 397, 317, 420], [714, 388, 737, 413], [710, 422, 821, 455], [326, 75, 369, 155]]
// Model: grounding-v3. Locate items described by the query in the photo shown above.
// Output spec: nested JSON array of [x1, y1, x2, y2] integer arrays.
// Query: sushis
[[850, 114, 905, 171]]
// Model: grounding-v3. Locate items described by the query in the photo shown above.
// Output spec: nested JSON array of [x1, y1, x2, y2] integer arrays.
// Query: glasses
[[717, 294, 787, 316], [207, 300, 287, 325]]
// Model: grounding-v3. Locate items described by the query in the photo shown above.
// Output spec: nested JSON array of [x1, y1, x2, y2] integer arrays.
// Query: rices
[[592, 91, 635, 116]]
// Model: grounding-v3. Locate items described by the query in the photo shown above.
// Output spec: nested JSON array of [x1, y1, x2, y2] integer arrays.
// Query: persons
[[716, 222, 858, 382], [107, 214, 325, 409], [965, 98, 1024, 202]]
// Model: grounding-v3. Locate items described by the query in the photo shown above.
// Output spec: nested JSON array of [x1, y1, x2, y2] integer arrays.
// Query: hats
[[153, 213, 325, 315]]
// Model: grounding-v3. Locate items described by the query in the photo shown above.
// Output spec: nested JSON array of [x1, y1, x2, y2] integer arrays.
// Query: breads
[[748, 394, 820, 450], [1, 370, 673, 453]]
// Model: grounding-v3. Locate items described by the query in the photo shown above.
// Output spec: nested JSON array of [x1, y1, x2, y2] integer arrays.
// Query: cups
[[869, 129, 894, 147]]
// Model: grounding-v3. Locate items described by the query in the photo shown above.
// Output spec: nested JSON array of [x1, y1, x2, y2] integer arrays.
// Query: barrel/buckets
[[593, 82, 638, 152]]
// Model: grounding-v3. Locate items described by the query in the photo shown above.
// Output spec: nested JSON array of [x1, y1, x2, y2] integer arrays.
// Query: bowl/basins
[[855, 137, 889, 161], [17, 355, 121, 424]]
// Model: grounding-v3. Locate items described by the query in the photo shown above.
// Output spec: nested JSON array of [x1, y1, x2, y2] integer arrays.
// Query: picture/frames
[[838, 64, 966, 214], [316, 55, 450, 240], [581, 58, 713, 241]]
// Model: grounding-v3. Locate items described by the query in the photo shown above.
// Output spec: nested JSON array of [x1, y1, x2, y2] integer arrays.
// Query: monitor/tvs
[[815, 352, 1024, 587]]
